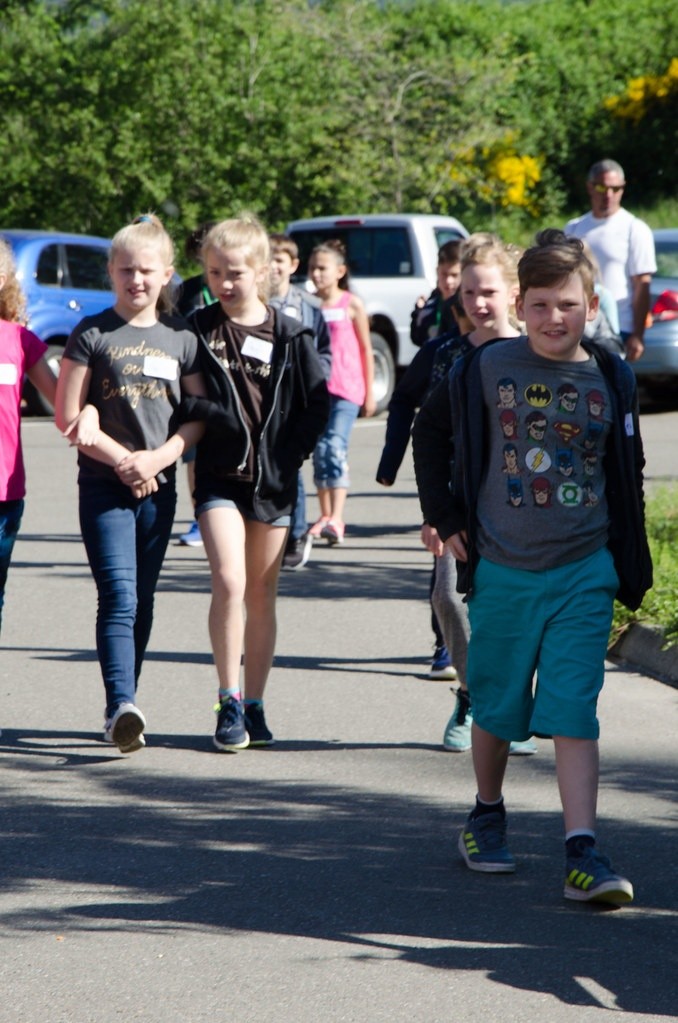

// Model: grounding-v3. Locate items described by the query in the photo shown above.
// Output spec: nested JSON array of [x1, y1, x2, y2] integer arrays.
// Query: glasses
[[590, 183, 622, 194]]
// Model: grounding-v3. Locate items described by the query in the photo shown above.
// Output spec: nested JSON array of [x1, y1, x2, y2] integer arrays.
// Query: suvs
[[0, 229, 188, 416]]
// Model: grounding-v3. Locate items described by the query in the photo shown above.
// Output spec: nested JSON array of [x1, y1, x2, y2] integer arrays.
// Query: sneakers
[[442, 686, 473, 752], [430, 646, 456, 681], [562, 839, 634, 906], [280, 532, 314, 570], [509, 737, 538, 755], [308, 520, 330, 538], [212, 700, 250, 750], [179, 519, 205, 547], [456, 807, 516, 874], [320, 519, 346, 545], [111, 703, 146, 755], [243, 707, 275, 747], [103, 707, 116, 743]]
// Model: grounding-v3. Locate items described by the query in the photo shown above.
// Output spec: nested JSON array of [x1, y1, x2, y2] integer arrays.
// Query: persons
[[304, 238, 376, 544], [563, 156, 660, 360], [177, 226, 219, 545], [179, 216, 335, 746], [377, 225, 655, 907], [55, 213, 206, 753], [0, 239, 100, 629], [267, 233, 333, 573]]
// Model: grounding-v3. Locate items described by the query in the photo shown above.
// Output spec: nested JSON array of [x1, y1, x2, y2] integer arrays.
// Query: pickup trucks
[[188, 214, 471, 417]]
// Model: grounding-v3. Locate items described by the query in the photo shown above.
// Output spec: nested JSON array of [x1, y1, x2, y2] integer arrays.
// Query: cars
[[620, 228, 678, 395]]
